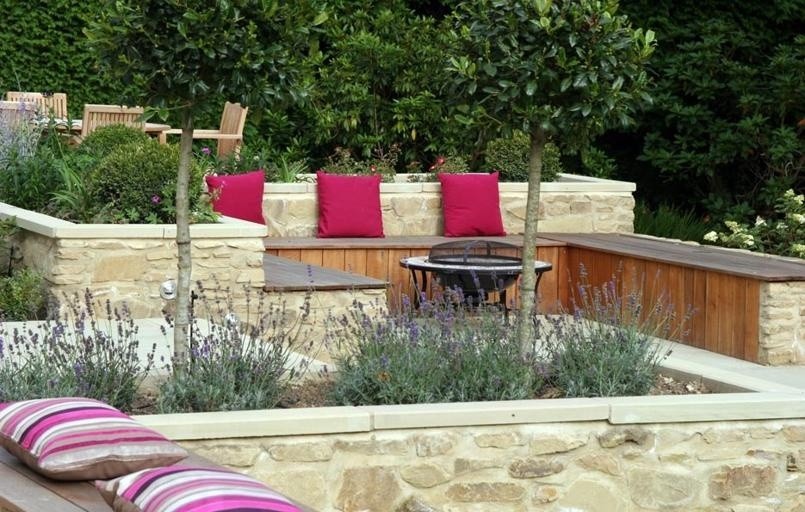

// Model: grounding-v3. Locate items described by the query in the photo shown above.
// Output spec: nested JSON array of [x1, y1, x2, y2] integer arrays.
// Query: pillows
[[0, 396, 188, 487], [84, 459, 308, 512], [436, 171, 506, 238], [313, 170, 384, 239], [204, 169, 266, 225]]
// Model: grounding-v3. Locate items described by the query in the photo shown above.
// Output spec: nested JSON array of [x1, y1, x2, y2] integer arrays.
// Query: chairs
[[77, 101, 144, 138], [163, 99, 250, 159], [0, 98, 43, 139], [7, 87, 74, 125]]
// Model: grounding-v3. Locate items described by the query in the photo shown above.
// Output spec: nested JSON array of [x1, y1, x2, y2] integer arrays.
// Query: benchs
[[1, 396, 314, 510], [522, 227, 805, 368], [256, 251, 394, 325], [258, 234, 565, 318]]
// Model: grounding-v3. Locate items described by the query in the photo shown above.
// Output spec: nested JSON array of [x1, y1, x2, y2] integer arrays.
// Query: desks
[[398, 253, 552, 334], [38, 114, 169, 146]]
